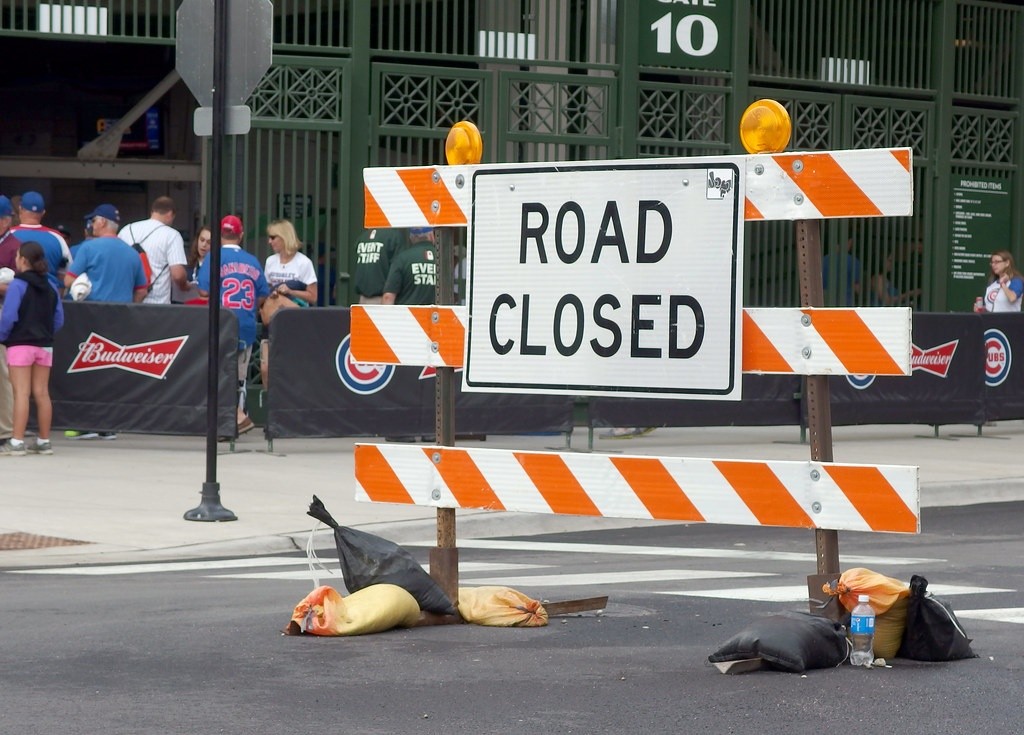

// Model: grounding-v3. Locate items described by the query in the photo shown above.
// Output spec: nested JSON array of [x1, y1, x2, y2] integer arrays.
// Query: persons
[[300, 240, 337, 307], [381, 227, 437, 307], [117, 195, 191, 306], [0, 195, 25, 444], [256, 218, 319, 391], [821, 226, 924, 316], [0, 240, 64, 454], [11, 194, 97, 302], [197, 215, 271, 442], [184, 222, 212, 307], [353, 227, 409, 306], [64, 202, 150, 440], [972, 249, 1024, 312]]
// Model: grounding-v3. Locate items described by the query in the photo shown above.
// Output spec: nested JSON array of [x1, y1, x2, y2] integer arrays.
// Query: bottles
[[850, 595, 876, 667]]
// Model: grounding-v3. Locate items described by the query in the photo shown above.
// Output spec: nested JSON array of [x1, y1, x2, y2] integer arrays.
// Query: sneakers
[[65, 430, 117, 440], [217, 417, 255, 442], [27, 441, 53, 455], [1, 439, 26, 456]]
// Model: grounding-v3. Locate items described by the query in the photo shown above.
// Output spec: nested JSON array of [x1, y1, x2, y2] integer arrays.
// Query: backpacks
[[127, 222, 168, 293]]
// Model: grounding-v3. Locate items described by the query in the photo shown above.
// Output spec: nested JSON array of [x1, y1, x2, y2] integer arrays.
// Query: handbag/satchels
[[259, 289, 301, 328]]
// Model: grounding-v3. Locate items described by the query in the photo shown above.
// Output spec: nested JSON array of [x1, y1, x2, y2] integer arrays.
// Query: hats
[[410, 227, 433, 234], [0, 195, 15, 218], [83, 204, 121, 225], [51, 223, 72, 238], [220, 216, 244, 234], [20, 191, 45, 212]]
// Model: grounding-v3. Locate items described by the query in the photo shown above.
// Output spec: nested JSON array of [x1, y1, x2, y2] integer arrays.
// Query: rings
[[283, 290, 285, 292]]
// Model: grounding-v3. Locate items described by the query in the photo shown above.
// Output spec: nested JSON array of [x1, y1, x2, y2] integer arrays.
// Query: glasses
[[989, 260, 1006, 266], [268, 235, 279, 240]]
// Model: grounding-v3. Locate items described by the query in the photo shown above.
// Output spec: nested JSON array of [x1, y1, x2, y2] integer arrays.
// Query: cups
[[974, 297, 984, 313]]
